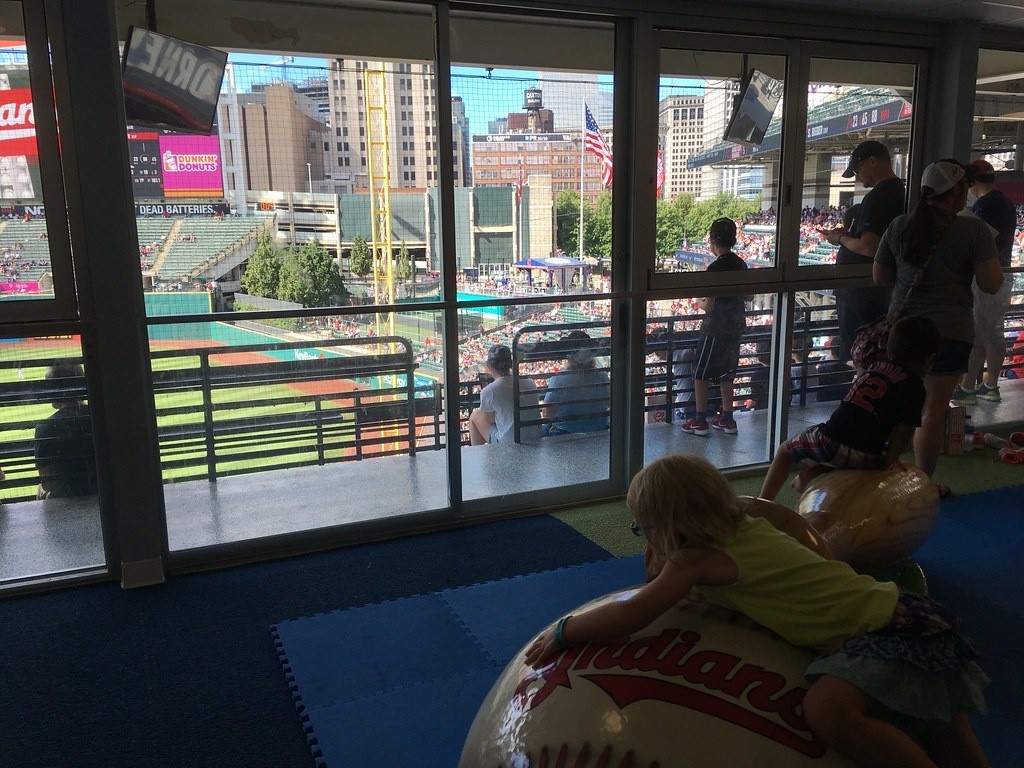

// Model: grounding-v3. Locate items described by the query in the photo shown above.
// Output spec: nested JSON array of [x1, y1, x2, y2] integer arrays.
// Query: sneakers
[[951, 384, 977, 406], [681, 417, 709, 435], [975, 383, 1001, 401], [710, 417, 738, 434]]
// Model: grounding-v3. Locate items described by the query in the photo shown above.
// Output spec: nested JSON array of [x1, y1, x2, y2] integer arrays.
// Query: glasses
[[630, 520, 645, 536], [851, 158, 869, 176], [957, 175, 974, 188]]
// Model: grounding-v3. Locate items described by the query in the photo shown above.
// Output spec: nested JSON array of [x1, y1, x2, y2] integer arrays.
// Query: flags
[[657, 149, 665, 198], [517, 161, 522, 206], [585, 105, 612, 189]]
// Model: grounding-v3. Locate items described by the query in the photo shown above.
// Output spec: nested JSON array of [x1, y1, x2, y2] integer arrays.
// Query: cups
[[549, 428, 571, 436]]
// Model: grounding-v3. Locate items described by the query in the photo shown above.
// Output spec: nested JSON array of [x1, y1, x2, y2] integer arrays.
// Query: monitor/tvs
[[723, 68, 784, 147], [121, 25, 229, 136]]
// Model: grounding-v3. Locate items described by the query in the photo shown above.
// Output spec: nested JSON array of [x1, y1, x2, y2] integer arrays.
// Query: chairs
[[689, 212, 841, 267], [418, 302, 614, 374]]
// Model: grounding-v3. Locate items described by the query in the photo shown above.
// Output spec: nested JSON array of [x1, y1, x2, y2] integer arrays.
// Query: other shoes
[[972, 431, 985, 450], [999, 447, 1024, 464], [934, 484, 951, 499], [984, 433, 1009, 450], [1010, 432, 1024, 446]]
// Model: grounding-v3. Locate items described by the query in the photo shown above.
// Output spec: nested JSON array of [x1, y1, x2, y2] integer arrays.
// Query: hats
[[841, 140, 891, 180], [965, 160, 998, 180], [921, 159, 970, 198], [560, 330, 590, 344], [710, 216, 737, 234]]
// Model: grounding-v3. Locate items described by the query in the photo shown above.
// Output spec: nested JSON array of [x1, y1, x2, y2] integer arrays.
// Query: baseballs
[[453, 581, 857, 768]]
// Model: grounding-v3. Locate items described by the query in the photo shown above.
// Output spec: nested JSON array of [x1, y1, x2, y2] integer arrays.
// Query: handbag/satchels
[[851, 304, 899, 371]]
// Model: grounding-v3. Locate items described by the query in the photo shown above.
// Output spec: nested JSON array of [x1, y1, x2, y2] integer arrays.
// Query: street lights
[[305, 162, 313, 193]]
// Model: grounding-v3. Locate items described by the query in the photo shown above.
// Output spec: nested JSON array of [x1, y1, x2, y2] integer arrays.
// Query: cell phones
[[816, 228, 828, 236]]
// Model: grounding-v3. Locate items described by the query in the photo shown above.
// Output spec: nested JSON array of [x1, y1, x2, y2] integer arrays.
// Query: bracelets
[[553, 615, 573, 645], [837, 235, 848, 246]]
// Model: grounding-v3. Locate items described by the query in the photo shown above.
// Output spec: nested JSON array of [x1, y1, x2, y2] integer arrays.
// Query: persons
[[33, 364, 97, 500], [873, 157, 1005, 497], [540, 330, 610, 438], [647, 328, 719, 420], [0, 209, 226, 279], [822, 140, 905, 396], [469, 344, 543, 446], [16, 369, 26, 379], [745, 336, 854, 410], [524, 451, 992, 768], [684, 202, 849, 262], [578, 273, 770, 333], [431, 276, 610, 388], [949, 159, 1017, 405], [293, 315, 431, 360], [756, 316, 940, 501]]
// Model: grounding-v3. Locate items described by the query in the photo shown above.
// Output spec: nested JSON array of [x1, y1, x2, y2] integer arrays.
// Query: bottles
[[542, 418, 552, 436], [964, 415, 974, 451]]
